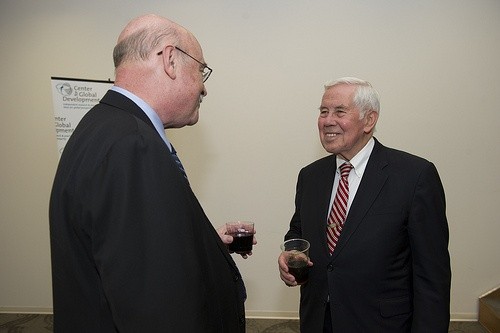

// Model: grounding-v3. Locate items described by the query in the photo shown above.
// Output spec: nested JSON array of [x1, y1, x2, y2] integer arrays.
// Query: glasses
[[157, 45, 212, 83]]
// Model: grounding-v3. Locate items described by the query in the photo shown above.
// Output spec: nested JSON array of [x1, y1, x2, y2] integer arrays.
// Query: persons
[[49, 13, 258, 333], [279, 76, 451, 333]]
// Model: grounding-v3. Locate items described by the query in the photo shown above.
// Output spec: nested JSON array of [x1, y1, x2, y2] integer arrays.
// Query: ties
[[170, 142, 191, 188], [327, 164, 353, 256]]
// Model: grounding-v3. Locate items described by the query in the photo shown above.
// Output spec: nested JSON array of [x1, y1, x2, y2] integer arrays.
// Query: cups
[[226, 222, 254, 253], [281, 239, 310, 285]]
